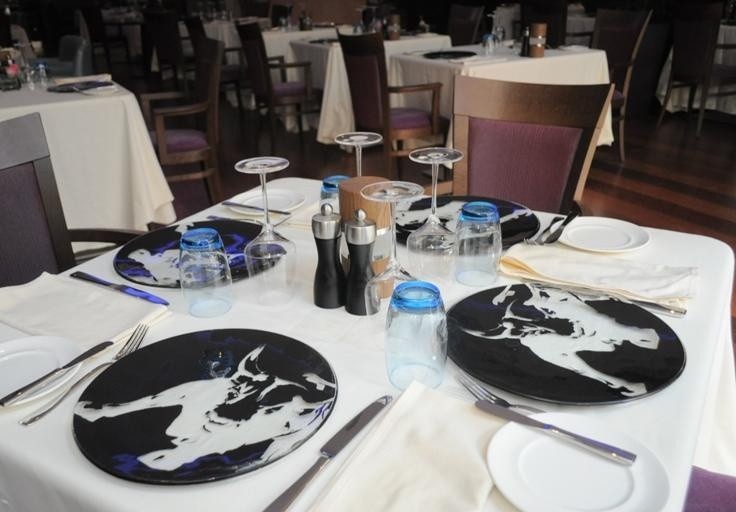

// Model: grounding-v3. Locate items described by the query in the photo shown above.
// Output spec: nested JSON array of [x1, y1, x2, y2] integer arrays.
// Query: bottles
[[0, 51, 20, 92], [518, 27, 530, 56], [177, 228, 235, 320], [454, 200, 503, 289], [191, 2, 232, 23], [343, 208, 379, 316], [307, 203, 346, 309], [384, 280, 450, 391], [318, 177, 351, 218]]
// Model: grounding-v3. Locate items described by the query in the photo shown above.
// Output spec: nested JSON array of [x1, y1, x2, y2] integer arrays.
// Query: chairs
[[684, 466, 736, 512]]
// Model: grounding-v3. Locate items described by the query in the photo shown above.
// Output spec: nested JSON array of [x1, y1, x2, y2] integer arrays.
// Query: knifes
[[545, 207, 580, 248], [70, 263, 170, 317], [474, 395, 636, 471], [265, 395, 394, 512], [218, 201, 293, 216], [72, 85, 107, 96], [1, 336, 114, 408]]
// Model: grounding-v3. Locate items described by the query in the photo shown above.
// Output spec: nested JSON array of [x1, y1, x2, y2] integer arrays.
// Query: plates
[[45, 81, 120, 95], [423, 51, 478, 59], [486, 412, 670, 512], [0, 333, 85, 408], [397, 194, 541, 255], [111, 218, 282, 290], [73, 327, 340, 486], [227, 187, 306, 214], [436, 280, 687, 402], [549, 213, 651, 255], [309, 37, 341, 45]]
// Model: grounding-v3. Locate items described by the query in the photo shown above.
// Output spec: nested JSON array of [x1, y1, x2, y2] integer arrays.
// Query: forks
[[456, 375, 562, 422], [525, 211, 565, 247], [11, 321, 151, 426]]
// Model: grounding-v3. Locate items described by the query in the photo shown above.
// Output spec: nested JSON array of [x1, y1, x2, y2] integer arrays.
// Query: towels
[[0, 271, 170, 358], [309, 379, 509, 512], [500, 244, 699, 308]]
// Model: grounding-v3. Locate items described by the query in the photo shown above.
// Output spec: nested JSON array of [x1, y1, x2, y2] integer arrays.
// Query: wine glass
[[238, 154, 298, 304], [487, 15, 506, 51], [404, 147, 465, 293], [330, 131, 384, 225], [361, 184, 422, 333], [16, 42, 52, 92]]
